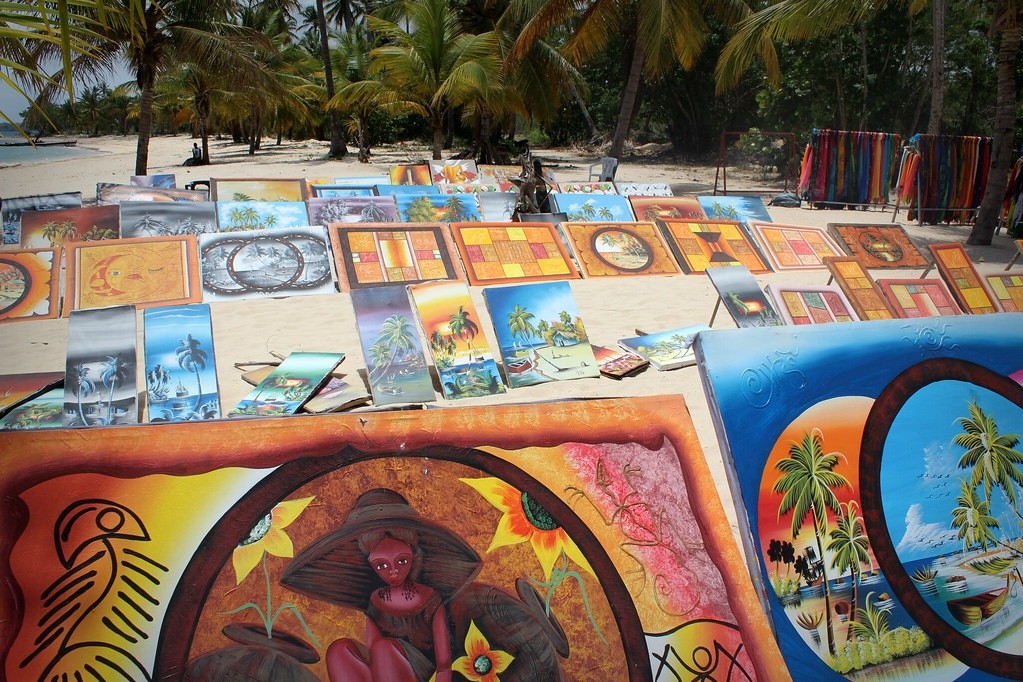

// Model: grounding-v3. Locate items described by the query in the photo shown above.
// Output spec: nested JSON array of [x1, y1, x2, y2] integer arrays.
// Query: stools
[[185, 179, 210, 191]]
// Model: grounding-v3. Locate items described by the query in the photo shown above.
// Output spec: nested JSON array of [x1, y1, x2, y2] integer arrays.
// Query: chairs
[[588, 157, 619, 182]]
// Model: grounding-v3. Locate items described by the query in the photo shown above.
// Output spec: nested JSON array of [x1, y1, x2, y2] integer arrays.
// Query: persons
[[182, 143, 202, 166]]
